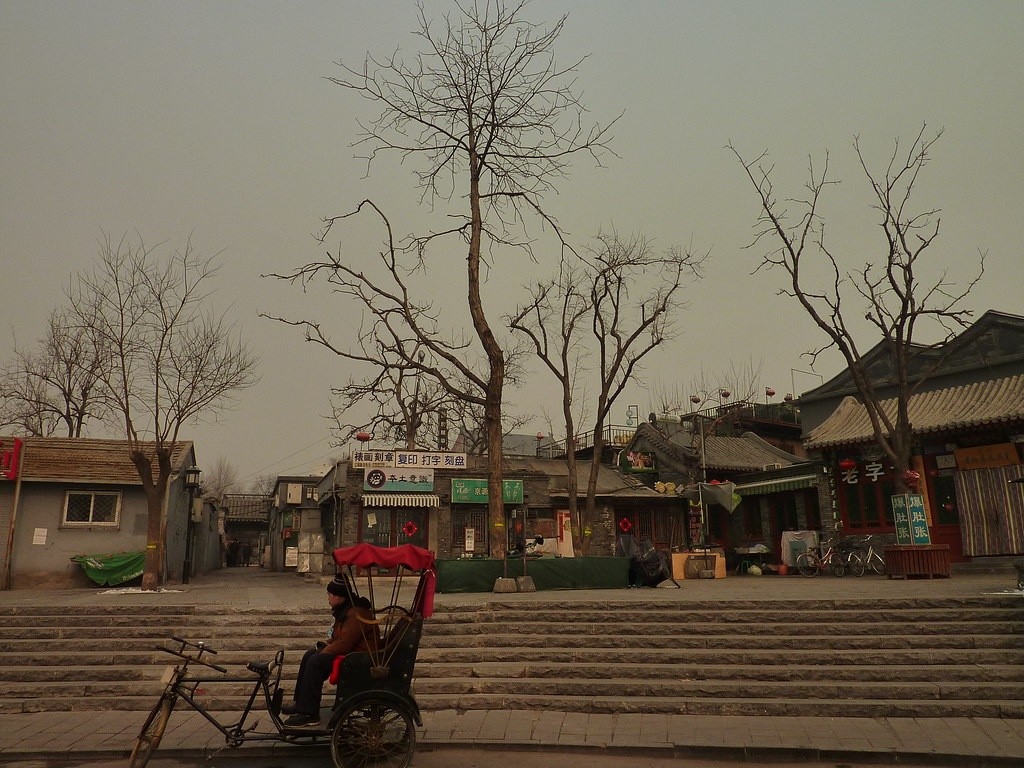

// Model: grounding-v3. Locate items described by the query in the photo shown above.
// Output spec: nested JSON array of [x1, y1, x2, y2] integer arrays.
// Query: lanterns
[[536, 433, 545, 440], [357, 431, 370, 443], [766, 388, 775, 397], [902, 468, 921, 488], [839, 457, 857, 478], [722, 389, 730, 399], [692, 395, 701, 404]]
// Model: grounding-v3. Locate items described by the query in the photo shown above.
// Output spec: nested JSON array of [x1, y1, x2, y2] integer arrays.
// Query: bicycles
[[796, 538, 845, 577], [847, 535, 886, 577]]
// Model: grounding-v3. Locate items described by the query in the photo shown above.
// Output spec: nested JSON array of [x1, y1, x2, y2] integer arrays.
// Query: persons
[[225, 538, 233, 567], [281, 572, 381, 728], [241, 541, 251, 567], [229, 539, 239, 567]]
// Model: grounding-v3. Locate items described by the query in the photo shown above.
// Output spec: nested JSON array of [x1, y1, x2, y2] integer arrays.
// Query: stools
[[741, 559, 752, 575]]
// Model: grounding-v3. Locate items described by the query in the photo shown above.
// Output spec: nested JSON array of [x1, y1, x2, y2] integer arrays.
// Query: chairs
[[344, 615, 408, 687]]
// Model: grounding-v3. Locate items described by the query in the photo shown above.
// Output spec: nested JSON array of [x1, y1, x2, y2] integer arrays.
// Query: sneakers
[[281, 704, 300, 716], [284, 714, 320, 727]]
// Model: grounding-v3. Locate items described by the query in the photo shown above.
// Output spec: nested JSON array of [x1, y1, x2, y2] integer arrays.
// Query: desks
[[671, 552, 727, 579]]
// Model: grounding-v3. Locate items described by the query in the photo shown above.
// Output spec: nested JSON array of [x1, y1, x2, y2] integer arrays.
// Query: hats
[[327, 573, 352, 598]]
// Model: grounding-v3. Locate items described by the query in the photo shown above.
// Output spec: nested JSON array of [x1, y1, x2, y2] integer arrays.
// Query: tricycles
[[130, 541, 437, 768]]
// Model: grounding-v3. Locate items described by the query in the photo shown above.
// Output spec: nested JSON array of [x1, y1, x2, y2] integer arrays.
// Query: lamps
[[623, 383, 799, 434]]
